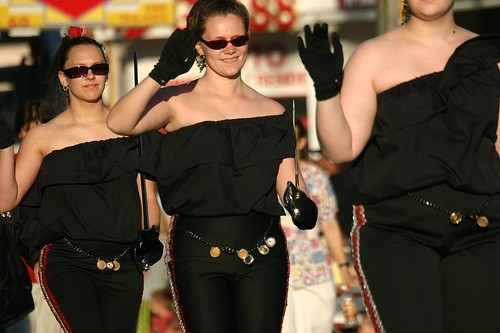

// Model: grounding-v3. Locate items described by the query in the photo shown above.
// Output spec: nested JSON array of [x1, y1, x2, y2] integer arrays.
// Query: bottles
[[341, 293, 359, 328]]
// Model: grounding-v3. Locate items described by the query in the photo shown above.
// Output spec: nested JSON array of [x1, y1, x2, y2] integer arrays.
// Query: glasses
[[62, 62, 109, 78], [200, 34, 249, 49]]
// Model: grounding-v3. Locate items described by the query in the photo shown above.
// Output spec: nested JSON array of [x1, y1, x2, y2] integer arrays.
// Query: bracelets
[[339, 262, 350, 267]]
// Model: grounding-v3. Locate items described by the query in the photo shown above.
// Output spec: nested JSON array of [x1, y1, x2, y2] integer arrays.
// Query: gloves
[[149, 28, 199, 85], [283, 185, 318, 230], [0, 112, 15, 149], [297, 21, 344, 100], [138, 224, 164, 265]]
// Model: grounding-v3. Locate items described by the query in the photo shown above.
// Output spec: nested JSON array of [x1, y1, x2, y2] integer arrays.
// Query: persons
[[0, 90, 67, 333], [277, 118, 357, 333], [0, 36, 164, 333], [106, 0, 318, 333], [296, 0, 500, 333]]
